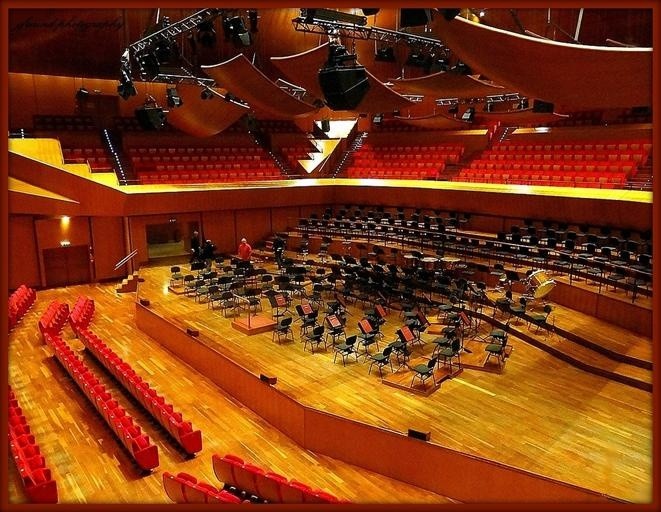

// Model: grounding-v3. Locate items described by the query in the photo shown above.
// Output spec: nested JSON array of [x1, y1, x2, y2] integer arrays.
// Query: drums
[[440, 257, 461, 271], [524, 270, 556, 298], [403, 254, 420, 268], [422, 257, 438, 270]]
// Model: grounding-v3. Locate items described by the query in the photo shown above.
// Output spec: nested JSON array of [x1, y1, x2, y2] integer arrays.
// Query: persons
[[201, 239, 216, 271], [189, 231, 201, 263], [239, 239, 251, 261], [272, 232, 283, 270], [145, 92, 158, 108]]
